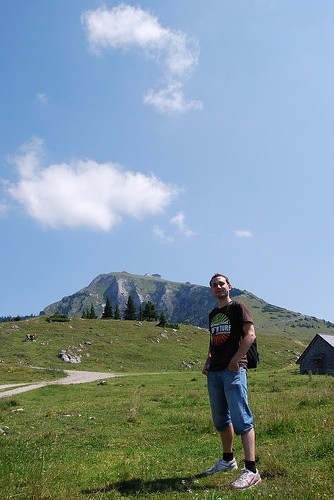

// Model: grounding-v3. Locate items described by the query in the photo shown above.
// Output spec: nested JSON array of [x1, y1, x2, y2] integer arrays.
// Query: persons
[[202, 273, 263, 489]]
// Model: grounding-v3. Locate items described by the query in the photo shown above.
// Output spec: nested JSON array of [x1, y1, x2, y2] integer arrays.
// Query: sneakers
[[204, 458, 238, 475], [230, 467, 262, 490]]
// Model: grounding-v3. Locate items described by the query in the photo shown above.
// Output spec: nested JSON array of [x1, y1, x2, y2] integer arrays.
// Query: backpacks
[[242, 330, 260, 369]]
